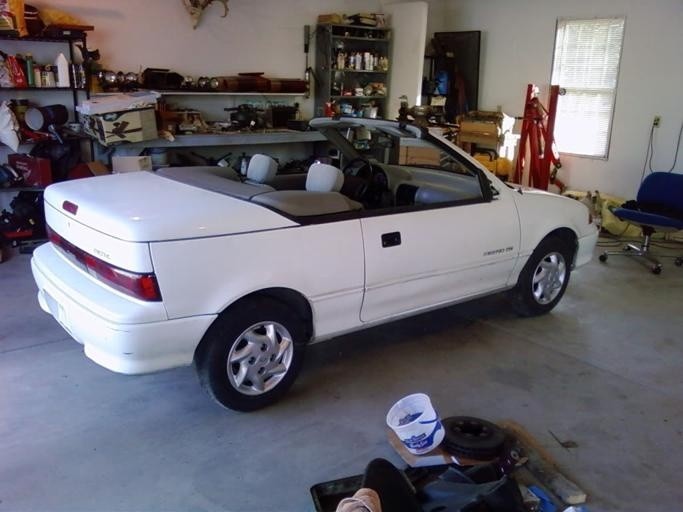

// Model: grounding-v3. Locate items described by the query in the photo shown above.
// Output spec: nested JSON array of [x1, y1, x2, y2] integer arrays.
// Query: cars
[[31, 117, 598, 411]]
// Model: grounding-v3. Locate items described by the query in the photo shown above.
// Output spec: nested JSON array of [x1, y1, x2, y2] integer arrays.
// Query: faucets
[[397, 101, 414, 130]]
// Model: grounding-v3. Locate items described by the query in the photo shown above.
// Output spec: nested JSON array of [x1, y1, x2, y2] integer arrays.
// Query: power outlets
[[652, 115, 661, 127]]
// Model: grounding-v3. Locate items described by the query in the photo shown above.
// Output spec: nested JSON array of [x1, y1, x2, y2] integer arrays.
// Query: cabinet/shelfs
[[91, 92, 327, 180], [0, 29, 96, 248], [314, 23, 392, 120], [422, 56, 456, 121]]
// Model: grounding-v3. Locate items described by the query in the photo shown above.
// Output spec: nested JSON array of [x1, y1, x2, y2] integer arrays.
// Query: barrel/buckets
[[386, 394, 445, 456], [54, 51, 71, 88]]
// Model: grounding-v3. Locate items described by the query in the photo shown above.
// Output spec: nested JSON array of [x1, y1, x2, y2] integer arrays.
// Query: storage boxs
[[460, 111, 515, 146], [396, 137, 441, 167]]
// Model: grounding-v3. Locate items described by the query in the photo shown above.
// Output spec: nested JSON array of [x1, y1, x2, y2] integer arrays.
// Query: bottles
[[335, 47, 379, 70], [24, 52, 34, 86]]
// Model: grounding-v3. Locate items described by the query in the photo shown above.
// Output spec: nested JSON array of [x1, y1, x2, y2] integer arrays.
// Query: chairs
[[599, 171, 683, 276], [304, 162, 364, 212], [244, 154, 278, 192]]
